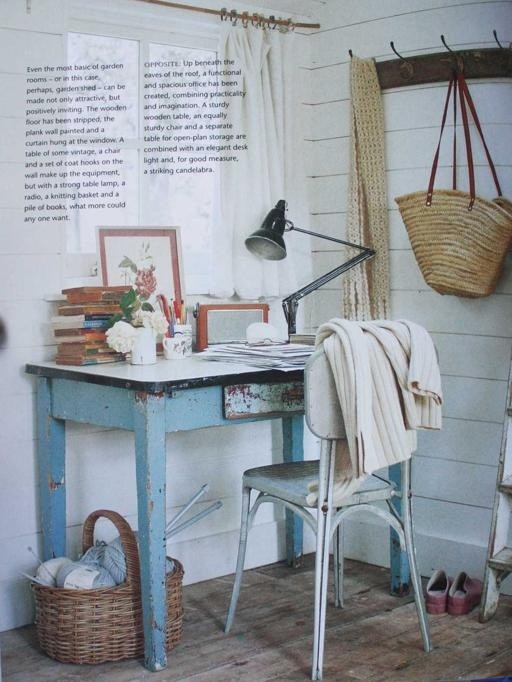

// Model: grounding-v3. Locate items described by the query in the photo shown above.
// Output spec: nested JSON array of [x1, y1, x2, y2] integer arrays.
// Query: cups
[[161, 333, 184, 360], [130, 325, 158, 366], [173, 323, 193, 357]]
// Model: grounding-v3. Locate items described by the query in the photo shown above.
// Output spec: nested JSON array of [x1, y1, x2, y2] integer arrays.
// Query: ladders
[[477, 369, 512, 625]]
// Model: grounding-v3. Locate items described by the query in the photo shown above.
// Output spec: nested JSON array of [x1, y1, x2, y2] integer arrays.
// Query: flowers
[[101, 287, 155, 355]]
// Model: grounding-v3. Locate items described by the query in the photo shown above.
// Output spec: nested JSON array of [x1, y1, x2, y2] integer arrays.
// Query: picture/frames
[[96, 228, 186, 360]]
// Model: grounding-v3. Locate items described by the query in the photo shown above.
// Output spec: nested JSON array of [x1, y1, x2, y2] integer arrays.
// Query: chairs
[[223, 323, 433, 682]]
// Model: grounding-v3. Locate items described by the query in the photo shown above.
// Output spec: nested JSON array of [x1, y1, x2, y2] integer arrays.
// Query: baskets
[[394, 189, 512, 298], [32, 510, 185, 664]]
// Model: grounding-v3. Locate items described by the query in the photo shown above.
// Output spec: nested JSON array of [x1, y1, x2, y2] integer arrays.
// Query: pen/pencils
[[159, 295, 186, 337]]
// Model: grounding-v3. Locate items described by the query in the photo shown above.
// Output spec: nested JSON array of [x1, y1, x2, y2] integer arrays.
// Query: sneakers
[[426, 568, 483, 615]]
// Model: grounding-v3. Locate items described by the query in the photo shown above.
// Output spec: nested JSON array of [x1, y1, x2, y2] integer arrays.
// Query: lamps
[[243, 199, 377, 346]]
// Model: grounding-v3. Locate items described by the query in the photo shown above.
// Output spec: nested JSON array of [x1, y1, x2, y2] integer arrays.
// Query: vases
[[128, 327, 157, 365]]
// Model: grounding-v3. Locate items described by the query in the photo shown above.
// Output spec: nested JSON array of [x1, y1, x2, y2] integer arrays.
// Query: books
[[52, 286, 134, 366]]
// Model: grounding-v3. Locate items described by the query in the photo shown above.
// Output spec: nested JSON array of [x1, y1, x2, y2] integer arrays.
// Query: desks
[[23, 359, 411, 670]]
